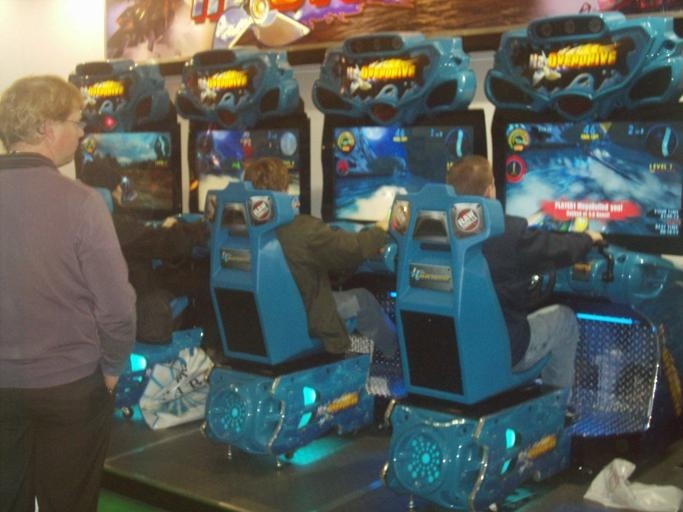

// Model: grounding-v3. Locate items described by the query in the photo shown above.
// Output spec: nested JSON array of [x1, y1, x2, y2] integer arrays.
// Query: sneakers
[[565, 406, 579, 424]]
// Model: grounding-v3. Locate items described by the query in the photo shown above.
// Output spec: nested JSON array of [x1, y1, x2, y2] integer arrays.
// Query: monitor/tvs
[[74, 123, 183, 221], [490, 108, 683, 257], [188, 120, 312, 223], [320, 110, 488, 225]]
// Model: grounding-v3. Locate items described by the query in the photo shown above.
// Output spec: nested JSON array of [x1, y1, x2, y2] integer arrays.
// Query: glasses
[[63, 117, 86, 129]]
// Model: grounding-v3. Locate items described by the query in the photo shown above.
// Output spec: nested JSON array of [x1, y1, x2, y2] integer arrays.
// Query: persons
[[444, 156, 604, 405], [77, 163, 204, 346], [0, 75, 138, 512], [242, 156, 400, 361]]
